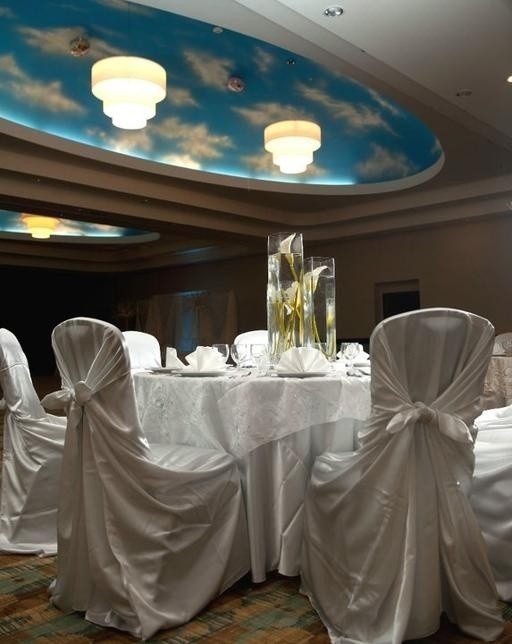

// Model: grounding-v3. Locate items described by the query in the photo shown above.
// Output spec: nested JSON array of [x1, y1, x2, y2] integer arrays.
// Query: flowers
[[270, 234, 333, 361]]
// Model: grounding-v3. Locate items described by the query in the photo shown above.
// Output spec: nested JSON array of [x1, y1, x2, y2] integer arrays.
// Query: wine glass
[[341, 342, 360, 375], [231, 344, 247, 372], [250, 344, 266, 376], [212, 344, 229, 363]]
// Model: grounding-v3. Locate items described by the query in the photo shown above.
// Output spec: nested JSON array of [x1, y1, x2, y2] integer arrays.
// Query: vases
[[266, 232, 338, 366]]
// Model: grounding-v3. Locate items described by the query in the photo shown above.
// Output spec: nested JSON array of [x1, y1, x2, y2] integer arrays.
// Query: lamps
[[23, 216, 59, 239], [263, 121, 321, 174], [91, 55, 167, 130]]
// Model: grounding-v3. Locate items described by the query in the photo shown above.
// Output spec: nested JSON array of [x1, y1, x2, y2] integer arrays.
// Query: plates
[[145, 367, 181, 374], [176, 371, 228, 376], [269, 371, 331, 377]]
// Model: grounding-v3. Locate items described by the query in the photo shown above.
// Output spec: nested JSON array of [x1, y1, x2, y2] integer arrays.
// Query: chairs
[[234, 330, 269, 367], [301, 307, 503, 642], [1, 329, 68, 556], [47, 317, 249, 640], [468, 401, 512, 604], [123, 331, 162, 371]]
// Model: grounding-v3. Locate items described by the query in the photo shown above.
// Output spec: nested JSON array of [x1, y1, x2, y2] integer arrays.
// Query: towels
[[185, 345, 227, 371], [275, 346, 333, 377]]
